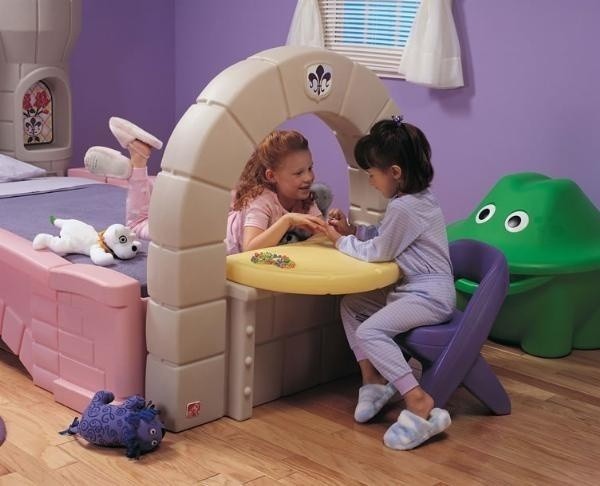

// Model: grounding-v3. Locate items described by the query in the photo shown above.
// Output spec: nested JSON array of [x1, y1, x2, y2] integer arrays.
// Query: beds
[[0, 0, 407, 434]]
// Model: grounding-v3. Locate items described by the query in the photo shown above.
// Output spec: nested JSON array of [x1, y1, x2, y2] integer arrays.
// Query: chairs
[[393, 239, 511, 416]]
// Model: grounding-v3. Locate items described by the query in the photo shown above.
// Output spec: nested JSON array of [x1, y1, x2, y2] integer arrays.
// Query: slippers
[[354, 383, 397, 422], [109, 117, 164, 150], [383, 407, 451, 450], [84, 145, 131, 178]]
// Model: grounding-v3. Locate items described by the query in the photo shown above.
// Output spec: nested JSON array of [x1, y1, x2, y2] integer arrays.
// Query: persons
[[326, 115, 457, 451], [85, 116, 327, 255]]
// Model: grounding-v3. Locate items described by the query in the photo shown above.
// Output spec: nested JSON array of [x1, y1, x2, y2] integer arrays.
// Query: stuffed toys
[[33, 216, 141, 266], [59, 391, 165, 459]]
